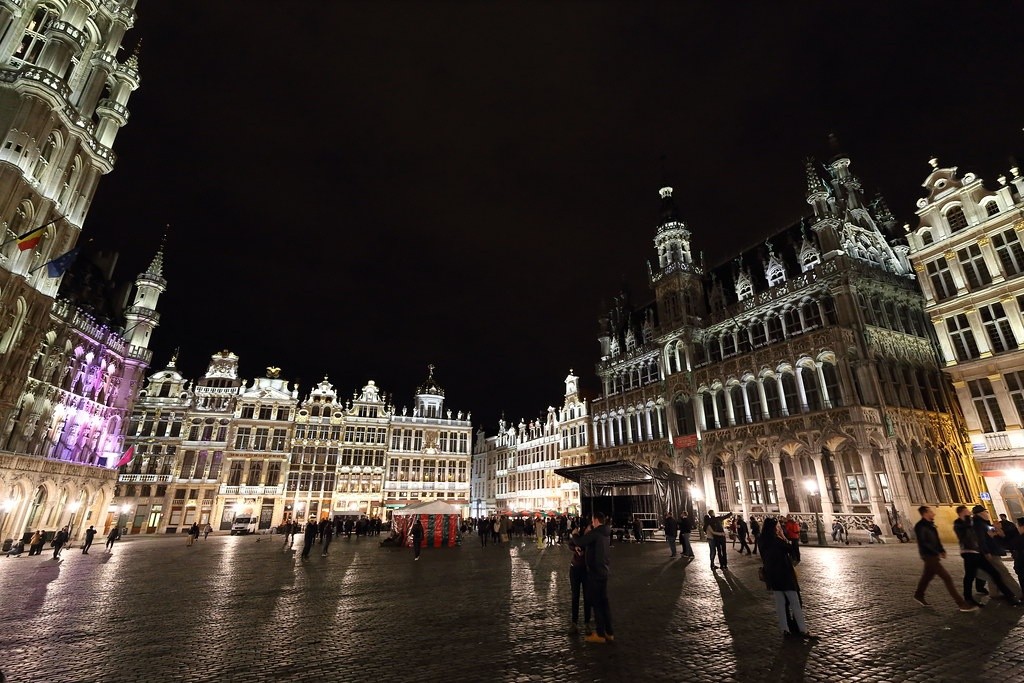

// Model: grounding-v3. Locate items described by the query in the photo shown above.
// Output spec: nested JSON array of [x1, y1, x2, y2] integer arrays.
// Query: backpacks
[[788, 521, 800, 533]]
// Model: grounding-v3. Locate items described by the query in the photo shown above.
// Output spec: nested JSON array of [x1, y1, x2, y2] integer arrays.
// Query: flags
[[16, 224, 47, 252], [48, 249, 75, 278], [117, 444, 134, 467]]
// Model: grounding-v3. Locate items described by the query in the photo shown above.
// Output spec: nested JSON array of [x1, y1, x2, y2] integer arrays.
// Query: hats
[[972, 505, 988, 514]]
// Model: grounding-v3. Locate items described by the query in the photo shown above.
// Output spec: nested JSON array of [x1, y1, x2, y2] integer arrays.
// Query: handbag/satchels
[[758, 566, 766, 582]]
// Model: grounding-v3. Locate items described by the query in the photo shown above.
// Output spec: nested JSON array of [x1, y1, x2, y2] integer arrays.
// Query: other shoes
[[604, 632, 614, 641], [745, 552, 751, 556], [783, 631, 792, 638], [801, 631, 818, 638], [914, 594, 932, 606], [977, 588, 989, 595], [322, 553, 329, 557], [585, 631, 605, 643], [688, 555, 694, 559], [965, 597, 985, 607], [680, 551, 687, 557], [959, 604, 980, 612], [711, 564, 719, 569], [994, 595, 1006, 600], [670, 555, 676, 559], [567, 624, 577, 635], [737, 550, 742, 554], [414, 556, 420, 561], [301, 558, 310, 563]]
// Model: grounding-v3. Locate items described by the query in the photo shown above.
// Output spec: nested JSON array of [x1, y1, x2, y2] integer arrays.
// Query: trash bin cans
[[800, 529, 809, 544], [2, 539, 13, 552]]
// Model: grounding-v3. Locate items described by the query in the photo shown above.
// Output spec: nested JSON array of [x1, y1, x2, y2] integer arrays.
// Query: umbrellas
[[493, 507, 571, 517]]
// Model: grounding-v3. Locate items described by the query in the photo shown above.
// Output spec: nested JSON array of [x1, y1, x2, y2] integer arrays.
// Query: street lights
[[296, 503, 301, 524], [0, 500, 16, 532], [691, 490, 705, 540], [805, 480, 827, 544], [68, 504, 77, 527]]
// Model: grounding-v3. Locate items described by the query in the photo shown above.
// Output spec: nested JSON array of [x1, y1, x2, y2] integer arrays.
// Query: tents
[[391, 499, 461, 547]]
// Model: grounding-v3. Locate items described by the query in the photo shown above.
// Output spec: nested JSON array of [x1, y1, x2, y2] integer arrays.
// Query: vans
[[332, 511, 368, 532], [230, 514, 258, 536]]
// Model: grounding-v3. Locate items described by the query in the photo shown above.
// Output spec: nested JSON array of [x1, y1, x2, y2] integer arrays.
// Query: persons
[[758, 518, 818, 637], [913, 506, 979, 612], [832, 520, 912, 544], [565, 511, 615, 643], [459, 514, 591, 550], [53, 525, 118, 560], [953, 505, 1024, 609], [7, 530, 46, 557], [379, 518, 424, 561], [663, 510, 695, 558], [605, 515, 643, 548], [702, 510, 808, 569], [186, 522, 211, 545], [279, 515, 392, 563]]
